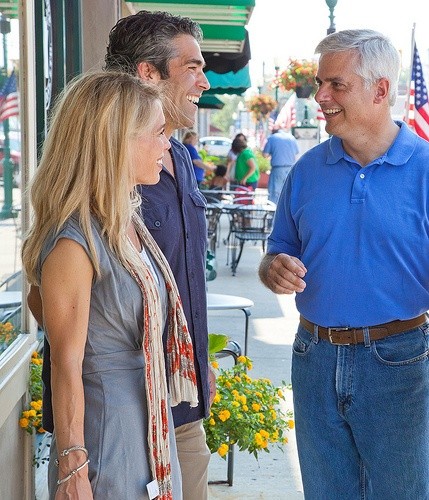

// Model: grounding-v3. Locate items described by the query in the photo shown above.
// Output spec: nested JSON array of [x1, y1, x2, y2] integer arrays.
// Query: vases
[[296, 87, 314, 98]]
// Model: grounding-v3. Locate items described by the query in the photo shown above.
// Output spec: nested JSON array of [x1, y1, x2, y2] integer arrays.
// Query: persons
[[27, 10, 211, 499], [20, 69, 199, 499], [182, 124, 300, 229], [258, 29, 428, 500]]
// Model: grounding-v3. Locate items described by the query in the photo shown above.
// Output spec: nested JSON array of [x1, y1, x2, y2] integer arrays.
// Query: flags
[[0, 70, 19, 123], [402, 37, 428, 142]]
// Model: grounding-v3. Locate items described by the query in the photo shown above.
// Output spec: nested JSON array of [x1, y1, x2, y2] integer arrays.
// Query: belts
[[299, 313, 427, 346]]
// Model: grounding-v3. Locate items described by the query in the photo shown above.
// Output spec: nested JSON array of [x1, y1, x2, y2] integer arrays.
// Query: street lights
[[272, 63, 280, 101], [0, 14, 19, 219]]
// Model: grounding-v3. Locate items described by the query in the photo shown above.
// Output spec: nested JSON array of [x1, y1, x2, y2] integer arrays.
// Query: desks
[[201, 189, 270, 196], [207, 202, 277, 216], [206, 292, 254, 374]]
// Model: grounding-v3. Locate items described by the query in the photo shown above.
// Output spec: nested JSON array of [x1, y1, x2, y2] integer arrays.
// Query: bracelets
[[54, 445, 91, 486]]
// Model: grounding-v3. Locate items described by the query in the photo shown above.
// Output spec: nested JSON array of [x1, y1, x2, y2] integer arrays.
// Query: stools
[[208, 341, 242, 487]]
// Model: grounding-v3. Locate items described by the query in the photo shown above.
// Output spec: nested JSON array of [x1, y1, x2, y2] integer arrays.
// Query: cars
[[0, 146, 21, 176], [198, 136, 233, 159]]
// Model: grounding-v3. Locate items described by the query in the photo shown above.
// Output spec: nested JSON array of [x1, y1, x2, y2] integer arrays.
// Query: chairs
[[205, 184, 276, 277]]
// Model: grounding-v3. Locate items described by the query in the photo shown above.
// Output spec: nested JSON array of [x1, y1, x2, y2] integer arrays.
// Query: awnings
[[121, 0, 251, 110]]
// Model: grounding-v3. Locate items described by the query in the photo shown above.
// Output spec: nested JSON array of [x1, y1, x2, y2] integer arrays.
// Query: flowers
[[19, 334, 295, 461], [246, 93, 278, 125], [267, 54, 319, 98], [0, 319, 21, 347]]
